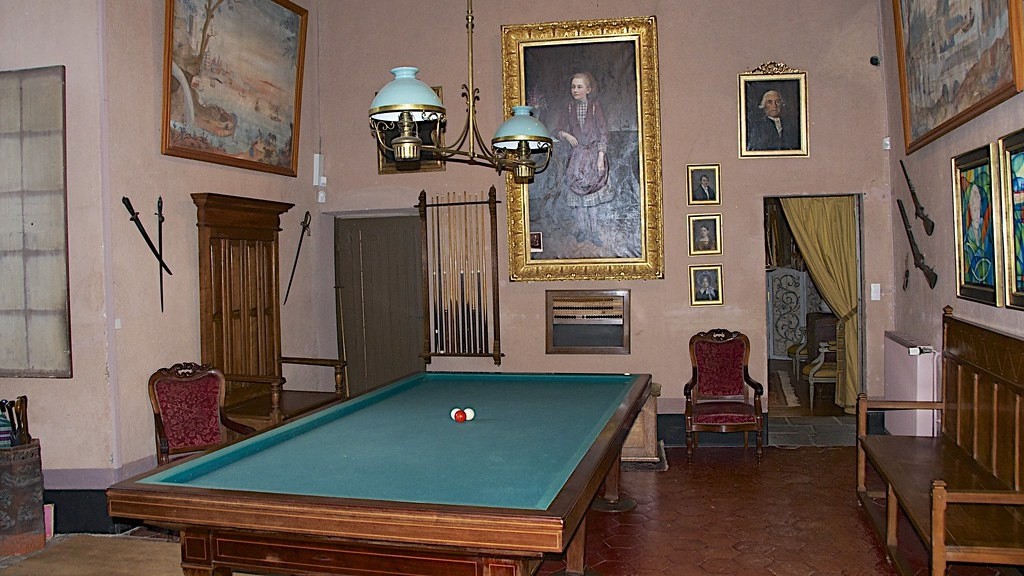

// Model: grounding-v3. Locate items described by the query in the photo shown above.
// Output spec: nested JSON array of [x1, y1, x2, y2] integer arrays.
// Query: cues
[[431, 190, 490, 354], [338, 287, 350, 397]]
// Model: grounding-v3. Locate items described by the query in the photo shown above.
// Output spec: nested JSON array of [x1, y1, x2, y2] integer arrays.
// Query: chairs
[[148, 192, 349, 541], [683, 331, 764, 464], [787, 327, 838, 412]]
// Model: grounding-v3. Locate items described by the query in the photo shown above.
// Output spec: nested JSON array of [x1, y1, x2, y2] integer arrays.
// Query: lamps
[[368, 2, 553, 180]]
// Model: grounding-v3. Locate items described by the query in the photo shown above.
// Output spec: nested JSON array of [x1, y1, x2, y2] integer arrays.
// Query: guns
[[899, 159, 935, 236], [897, 199, 938, 290]]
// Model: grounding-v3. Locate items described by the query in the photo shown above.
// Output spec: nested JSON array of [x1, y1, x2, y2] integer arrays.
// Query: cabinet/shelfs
[[620, 383, 660, 462], [805, 313, 839, 362]]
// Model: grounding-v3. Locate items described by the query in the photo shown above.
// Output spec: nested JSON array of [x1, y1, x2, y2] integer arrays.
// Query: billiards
[[454, 410, 466, 423], [451, 407, 462, 421], [464, 408, 474, 421]]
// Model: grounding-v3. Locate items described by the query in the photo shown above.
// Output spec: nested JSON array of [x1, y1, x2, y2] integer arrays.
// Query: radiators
[[884, 330, 943, 437]]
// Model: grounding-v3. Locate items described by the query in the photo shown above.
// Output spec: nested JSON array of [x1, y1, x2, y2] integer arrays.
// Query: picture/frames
[[162, 0, 309, 179], [501, 21, 810, 306], [891, 0, 1024, 313], [375, 85, 446, 175]]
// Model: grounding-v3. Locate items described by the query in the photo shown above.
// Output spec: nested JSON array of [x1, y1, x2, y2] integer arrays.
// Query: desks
[[105, 371, 651, 576]]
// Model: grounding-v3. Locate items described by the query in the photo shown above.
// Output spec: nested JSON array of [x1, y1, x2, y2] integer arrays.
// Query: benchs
[[855, 303, 1024, 576]]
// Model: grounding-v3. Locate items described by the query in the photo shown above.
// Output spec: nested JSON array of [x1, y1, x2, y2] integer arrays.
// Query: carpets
[[622, 439, 669, 473], [768, 370, 801, 408], [0, 532, 260, 576]]
